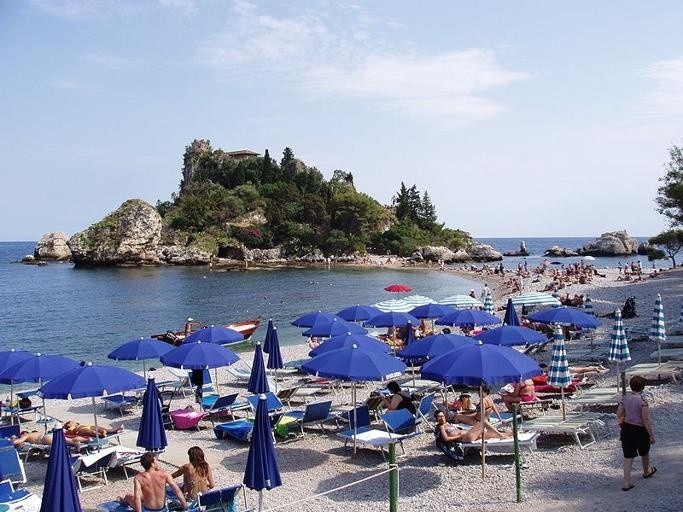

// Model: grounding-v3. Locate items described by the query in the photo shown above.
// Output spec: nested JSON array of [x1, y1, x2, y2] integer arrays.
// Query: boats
[[151, 315, 262, 346]]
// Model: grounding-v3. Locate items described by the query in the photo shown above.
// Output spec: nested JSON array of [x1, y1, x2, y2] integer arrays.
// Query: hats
[[188, 317, 194, 322], [459, 390, 471, 397]]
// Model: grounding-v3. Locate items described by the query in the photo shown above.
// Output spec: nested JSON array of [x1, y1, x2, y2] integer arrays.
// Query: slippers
[[642, 465, 657, 478], [621, 483, 635, 491]]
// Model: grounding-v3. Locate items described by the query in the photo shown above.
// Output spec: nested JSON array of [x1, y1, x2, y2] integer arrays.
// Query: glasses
[[437, 414, 444, 419]]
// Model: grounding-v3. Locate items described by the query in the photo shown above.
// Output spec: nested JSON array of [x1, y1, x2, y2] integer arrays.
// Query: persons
[[424, 255, 656, 303], [615, 374, 657, 491]]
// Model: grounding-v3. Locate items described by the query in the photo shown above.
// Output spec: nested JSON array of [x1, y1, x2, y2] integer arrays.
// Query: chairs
[[439, 329, 682, 473], [90, 360, 206, 421], [94, 475, 253, 511], [220, 329, 459, 405], [156, 382, 439, 460], [0, 388, 165, 510]]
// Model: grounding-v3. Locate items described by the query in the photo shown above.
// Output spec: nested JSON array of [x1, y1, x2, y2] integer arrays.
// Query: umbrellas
[[608, 307, 632, 395], [648, 291, 668, 365], [383, 284, 412, 300]]
